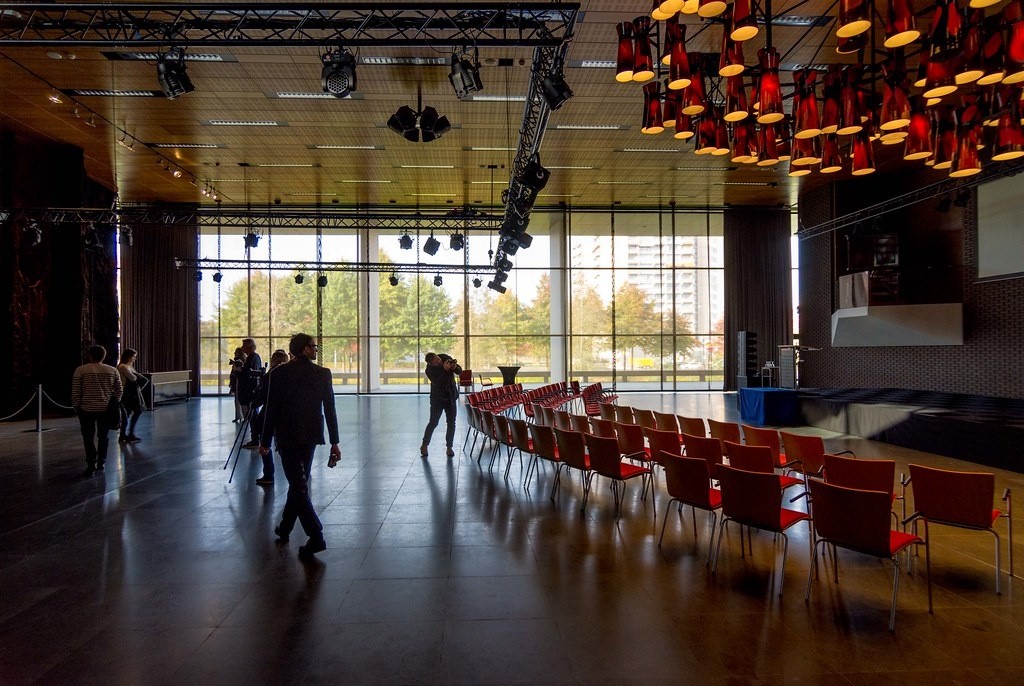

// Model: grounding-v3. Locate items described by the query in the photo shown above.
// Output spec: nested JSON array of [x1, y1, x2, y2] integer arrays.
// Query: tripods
[[222, 407, 255, 484]]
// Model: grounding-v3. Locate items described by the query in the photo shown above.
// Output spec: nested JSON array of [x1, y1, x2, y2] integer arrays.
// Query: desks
[[761, 366, 779, 388]]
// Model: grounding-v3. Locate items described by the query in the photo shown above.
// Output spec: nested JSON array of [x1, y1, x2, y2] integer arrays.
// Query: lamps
[[243, 226, 261, 251], [190, 268, 203, 282], [385, 93, 452, 144], [447, 44, 483, 100], [156, 45, 196, 101], [295, 268, 304, 284], [614, 0, 1024, 177], [320, 37, 358, 99], [389, 152, 551, 294], [18, 87, 216, 247], [534, 67, 576, 113], [213, 270, 222, 283], [318, 269, 327, 287]]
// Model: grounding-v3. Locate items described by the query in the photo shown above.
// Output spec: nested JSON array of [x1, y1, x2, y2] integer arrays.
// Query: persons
[[72, 345, 124, 477], [254, 352, 288, 483], [257, 332, 341, 555], [420, 352, 462, 457], [234, 338, 263, 449], [117, 348, 146, 442], [229, 347, 246, 423]]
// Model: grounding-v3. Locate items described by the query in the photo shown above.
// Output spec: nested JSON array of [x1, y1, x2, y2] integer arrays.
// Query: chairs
[[457, 370, 474, 398], [464, 381, 1015, 632], [479, 375, 494, 391]]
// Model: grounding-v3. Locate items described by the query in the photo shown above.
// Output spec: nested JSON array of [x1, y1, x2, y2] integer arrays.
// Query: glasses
[[311, 345, 319, 349], [132, 356, 138, 360]]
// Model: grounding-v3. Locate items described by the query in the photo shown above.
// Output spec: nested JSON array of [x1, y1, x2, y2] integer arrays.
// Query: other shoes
[[127, 436, 142, 442], [232, 418, 241, 423], [446, 449, 454, 457], [420, 445, 429, 456], [274, 525, 290, 542], [119, 435, 128, 441], [300, 540, 327, 555], [256, 478, 274, 483], [97, 466, 105, 476], [242, 442, 260, 449], [84, 467, 95, 475]]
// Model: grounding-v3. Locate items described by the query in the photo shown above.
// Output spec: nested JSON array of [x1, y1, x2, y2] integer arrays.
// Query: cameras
[[229, 359, 243, 366], [450, 359, 457, 364]]
